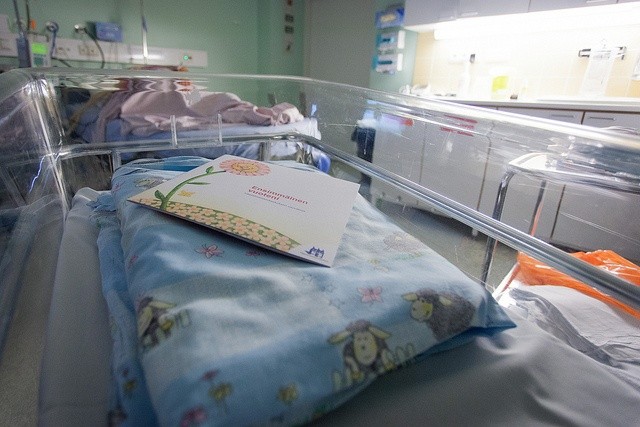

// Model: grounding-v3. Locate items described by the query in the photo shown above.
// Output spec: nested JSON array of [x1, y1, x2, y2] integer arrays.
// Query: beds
[[489, 254, 640, 379], [62, 101, 321, 161], [0, 70, 640, 425]]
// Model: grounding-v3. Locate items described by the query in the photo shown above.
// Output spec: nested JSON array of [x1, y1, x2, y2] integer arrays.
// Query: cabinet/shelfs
[[402, 1, 529, 33], [477, 105, 583, 244], [369, 108, 494, 239], [551, 111, 640, 263], [529, 0, 640, 15]]
